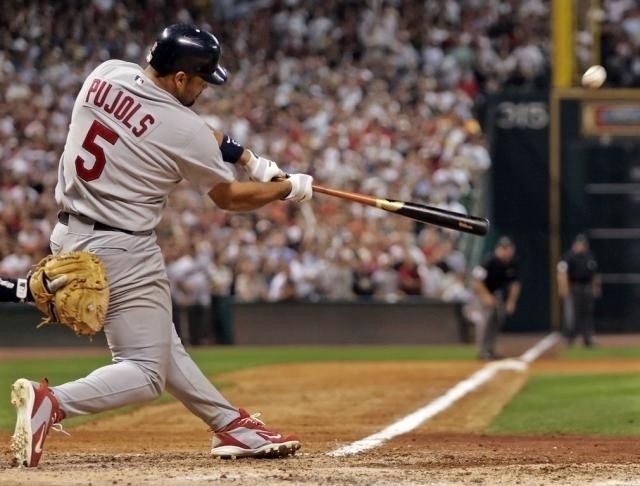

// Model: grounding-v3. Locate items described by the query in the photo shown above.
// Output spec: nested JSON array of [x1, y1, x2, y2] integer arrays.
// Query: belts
[[56, 207, 154, 239]]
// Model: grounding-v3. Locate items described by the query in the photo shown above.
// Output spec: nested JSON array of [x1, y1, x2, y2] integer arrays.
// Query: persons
[[486, 0, 640, 91], [6, 23, 316, 466], [1, 2, 486, 347], [473, 236, 526, 360], [0, 250, 112, 340], [555, 233, 604, 348]]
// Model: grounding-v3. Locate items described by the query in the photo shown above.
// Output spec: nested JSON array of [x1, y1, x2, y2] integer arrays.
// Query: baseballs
[[583, 65, 605, 88]]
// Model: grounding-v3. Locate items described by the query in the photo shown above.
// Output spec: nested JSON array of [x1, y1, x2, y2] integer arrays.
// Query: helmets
[[147, 21, 228, 88]]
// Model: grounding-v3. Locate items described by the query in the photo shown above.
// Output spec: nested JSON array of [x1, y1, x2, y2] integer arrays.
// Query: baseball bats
[[272, 176, 490, 237]]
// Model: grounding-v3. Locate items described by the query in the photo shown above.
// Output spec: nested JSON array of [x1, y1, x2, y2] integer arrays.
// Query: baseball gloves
[[29, 247, 111, 342]]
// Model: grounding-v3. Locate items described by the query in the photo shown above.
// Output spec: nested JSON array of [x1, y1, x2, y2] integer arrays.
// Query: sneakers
[[206, 408, 301, 462], [9, 375, 67, 470]]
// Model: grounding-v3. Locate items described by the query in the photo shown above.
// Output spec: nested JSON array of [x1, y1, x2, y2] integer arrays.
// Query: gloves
[[245, 147, 288, 185], [282, 174, 313, 204]]
